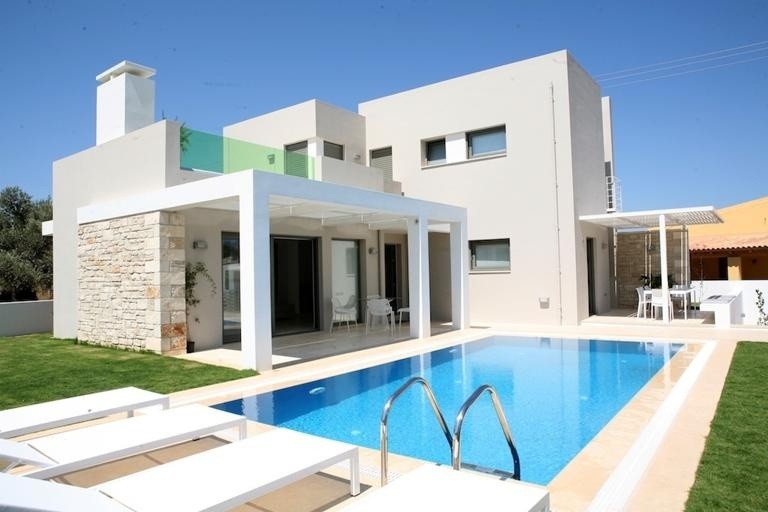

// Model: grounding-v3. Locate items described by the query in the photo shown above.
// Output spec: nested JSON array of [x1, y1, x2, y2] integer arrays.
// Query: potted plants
[[185, 258, 220, 353]]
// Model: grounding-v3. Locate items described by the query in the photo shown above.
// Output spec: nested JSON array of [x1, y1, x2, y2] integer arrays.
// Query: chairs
[[319, 466, 549, 511], [1, 427, 362, 510], [326, 294, 411, 339], [632, 282, 702, 325], [0, 385, 174, 440], [0, 405, 246, 479]]
